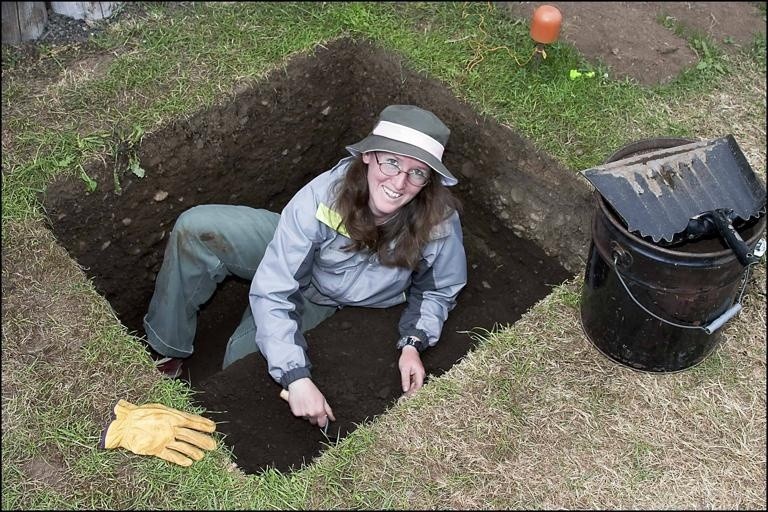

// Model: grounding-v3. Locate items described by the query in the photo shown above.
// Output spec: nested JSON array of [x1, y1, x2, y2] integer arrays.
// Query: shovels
[[579, 134, 767, 268], [279, 389, 358, 439]]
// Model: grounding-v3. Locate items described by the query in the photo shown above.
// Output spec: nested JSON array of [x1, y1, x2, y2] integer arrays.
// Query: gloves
[[102, 398, 216, 468]]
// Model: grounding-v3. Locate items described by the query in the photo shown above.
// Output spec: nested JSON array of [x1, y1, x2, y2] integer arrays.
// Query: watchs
[[395, 334, 424, 354]]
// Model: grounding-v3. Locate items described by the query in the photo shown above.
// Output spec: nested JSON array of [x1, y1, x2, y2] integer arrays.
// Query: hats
[[345, 104, 459, 186]]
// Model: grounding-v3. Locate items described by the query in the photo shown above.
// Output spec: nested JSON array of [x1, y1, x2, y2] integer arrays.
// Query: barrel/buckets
[[580, 137, 767, 372]]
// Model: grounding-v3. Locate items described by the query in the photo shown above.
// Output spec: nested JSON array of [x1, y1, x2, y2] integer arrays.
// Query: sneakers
[[152, 354, 182, 378]]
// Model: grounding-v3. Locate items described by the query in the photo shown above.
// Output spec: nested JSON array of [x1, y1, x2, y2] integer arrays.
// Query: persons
[[142, 104, 468, 428]]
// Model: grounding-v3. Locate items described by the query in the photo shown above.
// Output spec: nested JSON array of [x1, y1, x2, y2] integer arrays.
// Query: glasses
[[374, 151, 433, 188]]
